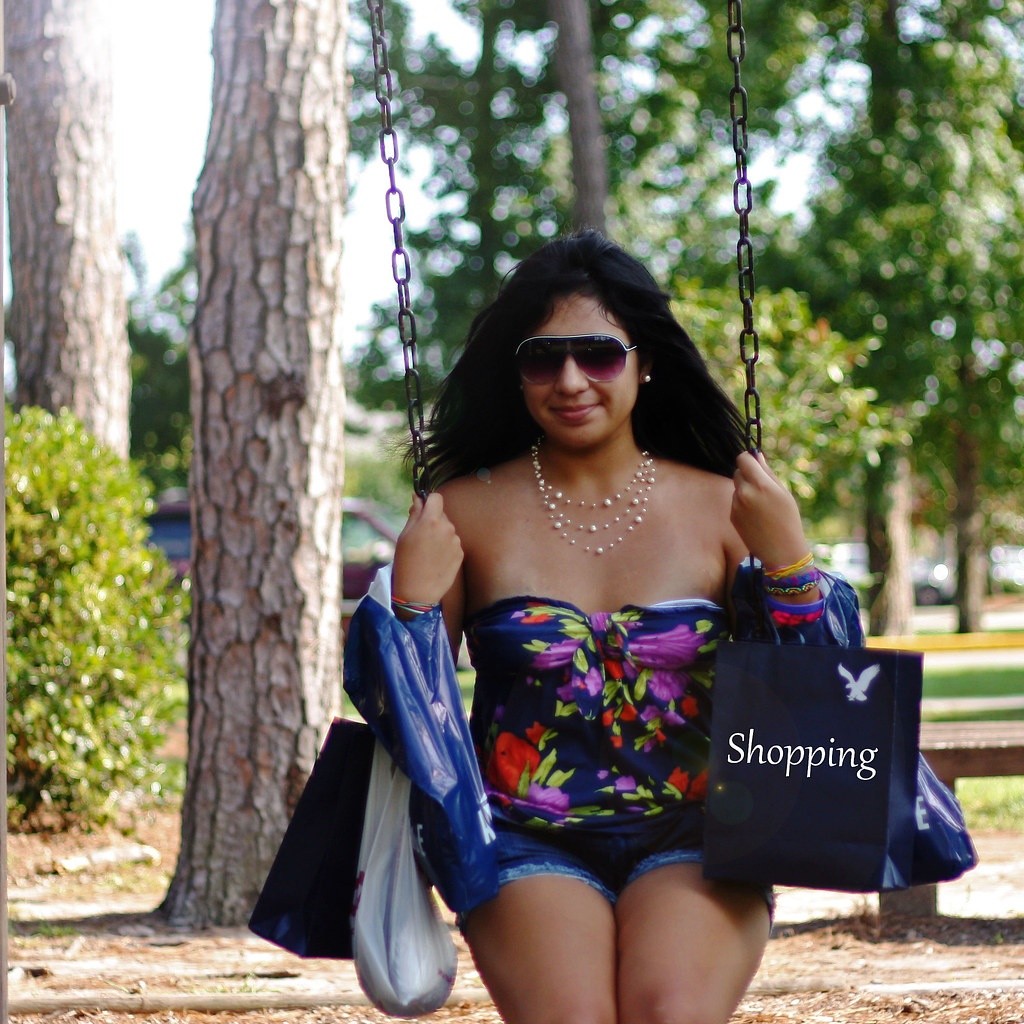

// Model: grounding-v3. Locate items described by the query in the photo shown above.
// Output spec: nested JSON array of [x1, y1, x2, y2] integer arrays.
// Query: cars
[[144, 500, 398, 633], [815, 544, 956, 607]]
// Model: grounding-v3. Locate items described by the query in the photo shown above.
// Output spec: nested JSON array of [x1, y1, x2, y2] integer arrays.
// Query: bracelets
[[762, 553, 820, 597], [391, 598, 440, 617]]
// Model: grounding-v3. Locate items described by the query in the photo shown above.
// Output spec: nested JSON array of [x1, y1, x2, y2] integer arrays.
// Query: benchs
[[878, 721, 1024, 917]]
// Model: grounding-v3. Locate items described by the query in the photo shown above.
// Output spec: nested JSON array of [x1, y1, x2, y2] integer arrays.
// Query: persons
[[360, 225, 864, 1024]]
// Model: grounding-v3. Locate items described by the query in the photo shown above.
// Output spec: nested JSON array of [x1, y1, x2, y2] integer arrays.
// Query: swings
[[366, 0, 764, 574]]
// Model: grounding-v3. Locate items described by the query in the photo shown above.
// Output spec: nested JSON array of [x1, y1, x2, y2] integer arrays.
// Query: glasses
[[514, 334, 637, 385]]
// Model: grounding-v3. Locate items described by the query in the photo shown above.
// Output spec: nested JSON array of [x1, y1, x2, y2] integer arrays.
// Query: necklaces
[[531, 432, 656, 554]]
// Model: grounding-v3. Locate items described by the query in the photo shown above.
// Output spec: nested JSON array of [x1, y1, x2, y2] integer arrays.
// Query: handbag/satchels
[[249, 561, 500, 1019], [701, 557, 978, 894]]
